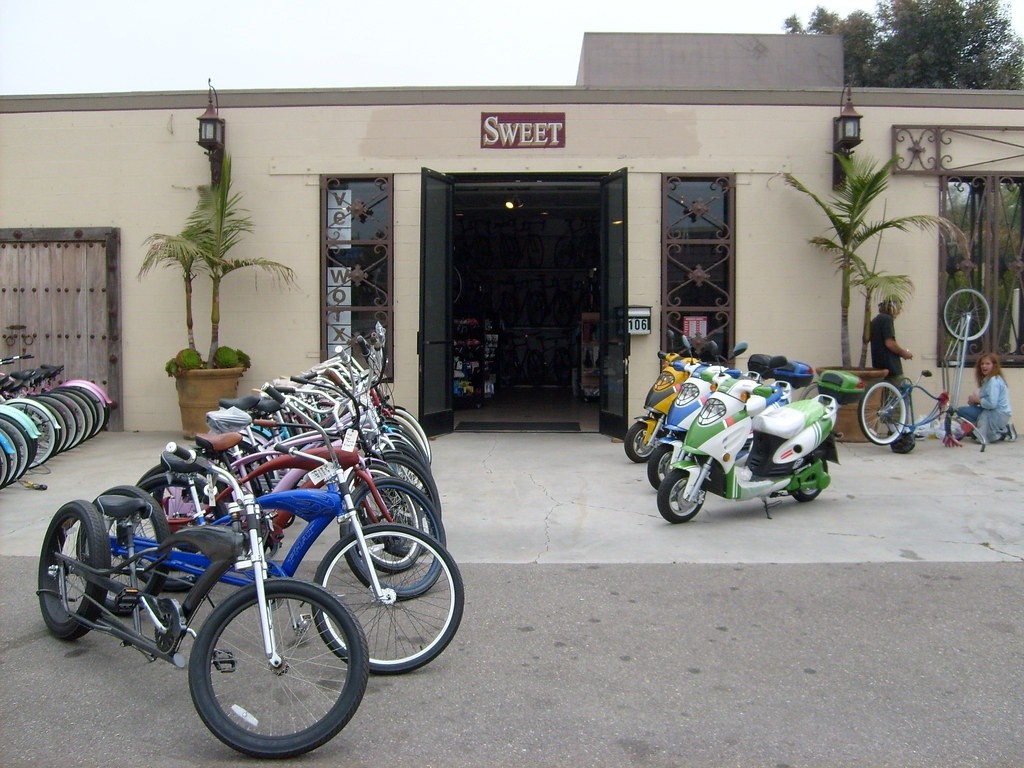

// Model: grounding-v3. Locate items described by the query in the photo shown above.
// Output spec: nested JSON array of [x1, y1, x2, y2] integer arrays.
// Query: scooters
[[624, 334, 865, 524]]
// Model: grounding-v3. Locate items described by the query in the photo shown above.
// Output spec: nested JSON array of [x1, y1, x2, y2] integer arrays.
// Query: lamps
[[832, 71, 864, 191], [197, 78, 226, 189]]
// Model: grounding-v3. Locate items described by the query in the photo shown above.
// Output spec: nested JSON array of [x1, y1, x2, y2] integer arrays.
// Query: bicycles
[[856, 289, 991, 452], [504, 330, 574, 388], [0, 354, 113, 491], [39, 324, 465, 763], [448, 212, 605, 327]]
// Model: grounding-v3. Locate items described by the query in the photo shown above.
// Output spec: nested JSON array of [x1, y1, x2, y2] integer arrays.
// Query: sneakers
[[1008, 423, 1017, 442]]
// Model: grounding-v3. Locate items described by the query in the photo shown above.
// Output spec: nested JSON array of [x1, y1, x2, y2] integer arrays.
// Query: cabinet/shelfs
[[452, 312, 501, 403], [484, 268, 590, 388]]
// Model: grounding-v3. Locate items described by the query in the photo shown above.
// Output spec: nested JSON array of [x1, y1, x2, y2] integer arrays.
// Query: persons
[[957, 352, 1017, 443], [871, 294, 927, 441]]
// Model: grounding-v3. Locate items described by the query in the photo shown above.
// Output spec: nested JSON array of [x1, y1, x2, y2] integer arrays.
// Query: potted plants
[[133, 153, 302, 439], [783, 150, 972, 443]]
